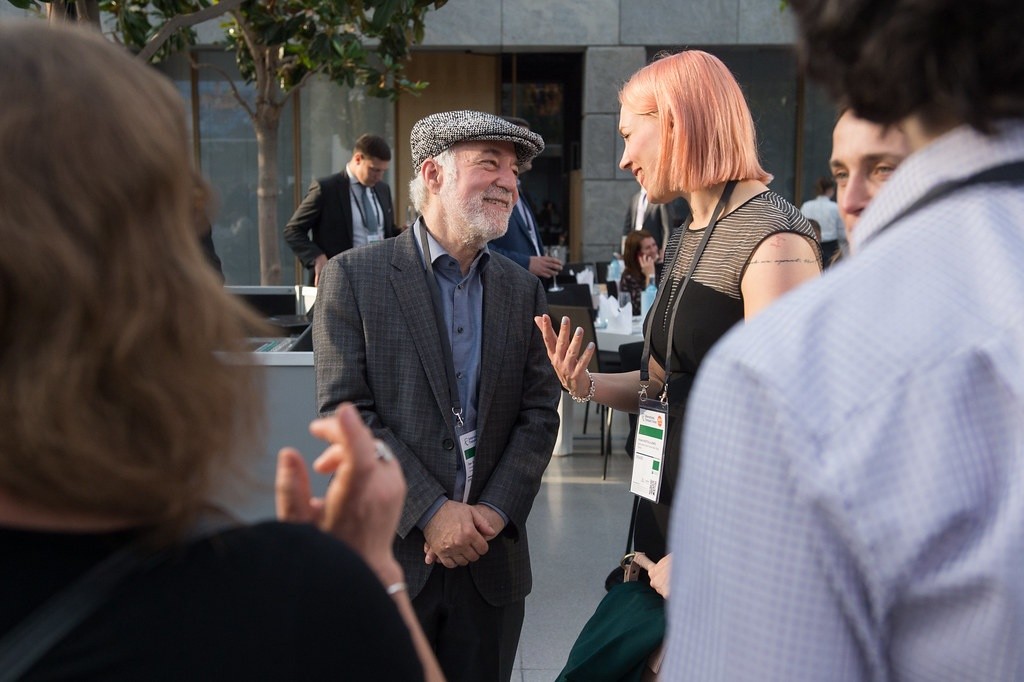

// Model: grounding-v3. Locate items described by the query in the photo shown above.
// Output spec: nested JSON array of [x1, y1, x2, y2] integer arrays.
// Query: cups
[[590, 291, 600, 321], [585, 265, 598, 291], [618, 292, 631, 312]]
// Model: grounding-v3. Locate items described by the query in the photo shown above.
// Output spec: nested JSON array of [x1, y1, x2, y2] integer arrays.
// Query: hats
[[410, 110, 545, 169]]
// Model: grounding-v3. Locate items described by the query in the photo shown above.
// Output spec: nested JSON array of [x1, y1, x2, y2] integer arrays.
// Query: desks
[[586, 315, 651, 453], [574, 282, 621, 310]]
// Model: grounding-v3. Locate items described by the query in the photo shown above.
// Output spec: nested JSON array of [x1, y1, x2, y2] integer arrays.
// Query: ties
[[362, 186, 378, 235], [635, 194, 646, 231]]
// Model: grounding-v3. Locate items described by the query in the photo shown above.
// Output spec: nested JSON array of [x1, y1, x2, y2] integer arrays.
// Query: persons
[[0, 20, 448, 682], [487, 116, 564, 292], [311, 110, 562, 682], [283, 133, 408, 323], [534, 0, 1023, 681]]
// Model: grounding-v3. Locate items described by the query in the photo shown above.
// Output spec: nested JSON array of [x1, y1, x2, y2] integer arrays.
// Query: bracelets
[[386, 582, 407, 596], [568, 368, 595, 403]]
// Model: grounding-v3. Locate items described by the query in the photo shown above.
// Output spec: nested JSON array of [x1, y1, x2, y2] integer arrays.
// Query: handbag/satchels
[[605, 560, 631, 592], [616, 552, 656, 682]]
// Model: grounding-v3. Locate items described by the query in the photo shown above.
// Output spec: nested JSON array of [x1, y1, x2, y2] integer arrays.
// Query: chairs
[[536, 255, 664, 481]]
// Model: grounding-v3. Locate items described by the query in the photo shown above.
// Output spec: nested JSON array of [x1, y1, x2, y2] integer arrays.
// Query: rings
[[374, 438, 391, 462]]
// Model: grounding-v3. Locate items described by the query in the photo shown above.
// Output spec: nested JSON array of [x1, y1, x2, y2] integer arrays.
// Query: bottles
[[644, 278, 658, 316], [610, 254, 619, 280]]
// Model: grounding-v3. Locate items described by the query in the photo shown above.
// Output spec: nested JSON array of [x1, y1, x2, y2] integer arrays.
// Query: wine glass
[[544, 246, 566, 292]]
